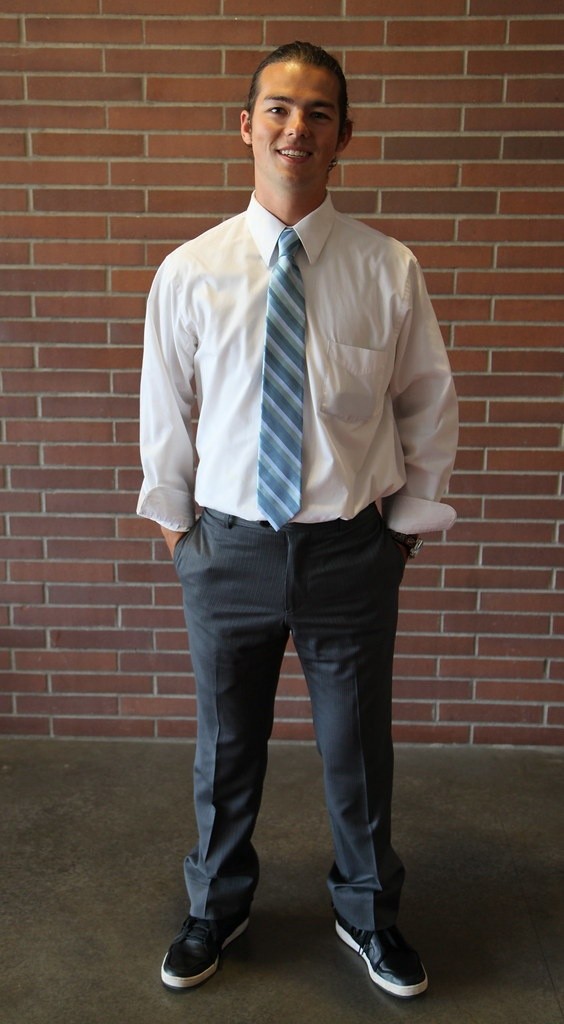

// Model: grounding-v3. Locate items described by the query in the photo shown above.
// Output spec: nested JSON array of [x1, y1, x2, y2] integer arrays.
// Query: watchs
[[389, 530, 424, 560]]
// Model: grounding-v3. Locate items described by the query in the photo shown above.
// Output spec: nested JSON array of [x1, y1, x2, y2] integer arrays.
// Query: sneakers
[[331, 901, 429, 999], [160, 896, 254, 989]]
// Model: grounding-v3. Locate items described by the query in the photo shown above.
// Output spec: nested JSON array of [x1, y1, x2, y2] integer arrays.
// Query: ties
[[256, 229, 306, 532]]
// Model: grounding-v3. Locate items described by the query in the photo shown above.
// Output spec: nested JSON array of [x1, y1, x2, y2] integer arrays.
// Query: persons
[[135, 39, 461, 1001]]
